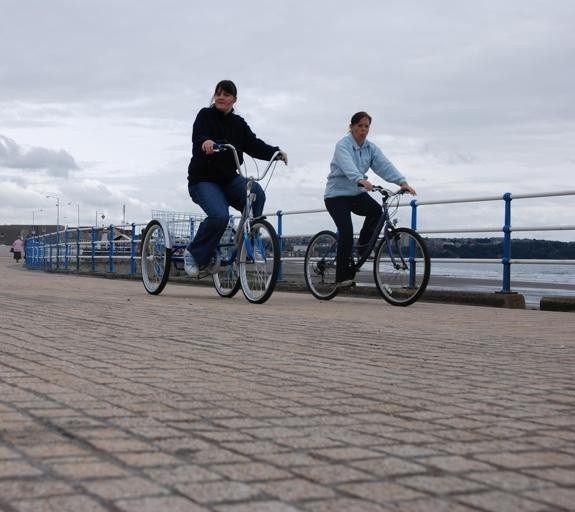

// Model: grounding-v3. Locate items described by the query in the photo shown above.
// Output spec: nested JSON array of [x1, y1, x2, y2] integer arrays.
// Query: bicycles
[[304, 185, 430, 305]]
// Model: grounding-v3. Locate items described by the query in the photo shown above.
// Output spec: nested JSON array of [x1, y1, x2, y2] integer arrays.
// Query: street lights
[[47, 196, 59, 232], [96, 211, 105, 227], [68, 203, 79, 226], [33, 210, 43, 236]]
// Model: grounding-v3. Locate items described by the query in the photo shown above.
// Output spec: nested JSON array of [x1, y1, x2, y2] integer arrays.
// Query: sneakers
[[183, 248, 200, 277], [356, 240, 375, 258], [336, 280, 356, 288]]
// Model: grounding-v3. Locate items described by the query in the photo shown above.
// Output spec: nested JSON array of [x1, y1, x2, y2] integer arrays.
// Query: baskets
[[151, 209, 234, 257]]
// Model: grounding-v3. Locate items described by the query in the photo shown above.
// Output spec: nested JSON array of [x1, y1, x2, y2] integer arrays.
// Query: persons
[[13, 235, 23, 263], [324, 112, 417, 289], [182, 80, 288, 276]]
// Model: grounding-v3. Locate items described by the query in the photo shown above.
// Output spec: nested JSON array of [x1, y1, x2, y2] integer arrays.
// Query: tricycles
[[141, 144, 280, 304]]
